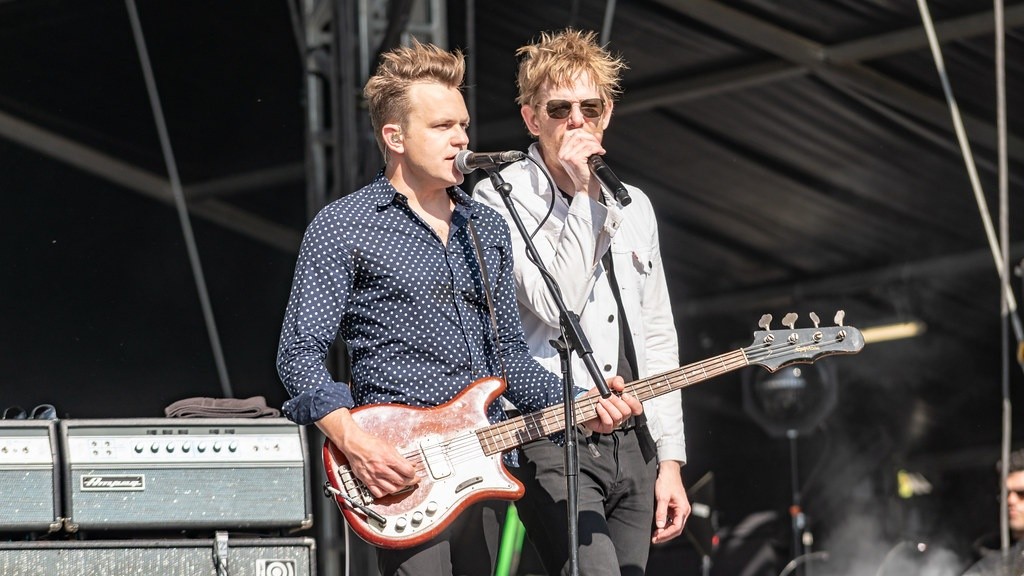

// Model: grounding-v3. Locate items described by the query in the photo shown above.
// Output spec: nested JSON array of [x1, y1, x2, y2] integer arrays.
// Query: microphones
[[587, 153, 631, 206], [453, 150, 526, 174]]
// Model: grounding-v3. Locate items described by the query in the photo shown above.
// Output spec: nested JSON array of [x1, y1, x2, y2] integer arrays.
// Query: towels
[[164, 396, 280, 418]]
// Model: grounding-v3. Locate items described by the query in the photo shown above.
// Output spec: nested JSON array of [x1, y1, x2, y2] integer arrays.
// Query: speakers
[[0, 531, 318, 576]]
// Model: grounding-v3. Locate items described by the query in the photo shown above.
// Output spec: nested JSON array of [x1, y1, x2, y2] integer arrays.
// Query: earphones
[[392, 131, 400, 143]]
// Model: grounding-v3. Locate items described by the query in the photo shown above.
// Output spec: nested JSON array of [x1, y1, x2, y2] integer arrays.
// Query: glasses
[[1007, 490, 1024, 500], [536, 98, 603, 119]]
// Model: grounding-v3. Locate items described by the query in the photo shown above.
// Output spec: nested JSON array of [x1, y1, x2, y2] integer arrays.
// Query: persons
[[959, 450, 1024, 576], [274, 28, 691, 576]]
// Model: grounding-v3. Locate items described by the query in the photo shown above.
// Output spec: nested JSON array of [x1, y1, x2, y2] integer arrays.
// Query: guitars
[[318, 305, 868, 556]]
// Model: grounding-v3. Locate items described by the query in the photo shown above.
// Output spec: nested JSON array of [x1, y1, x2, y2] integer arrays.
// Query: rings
[[614, 421, 623, 427]]
